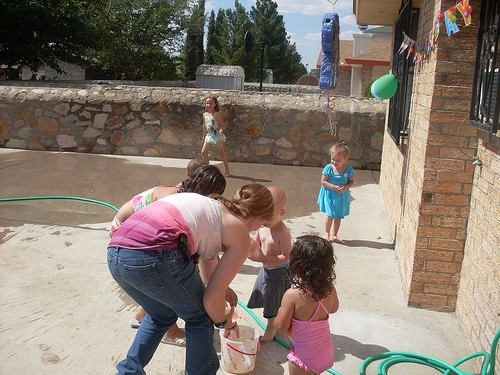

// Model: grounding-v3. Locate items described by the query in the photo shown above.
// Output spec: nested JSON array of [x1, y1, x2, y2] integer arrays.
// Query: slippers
[[129, 317, 142, 328], [160, 332, 187, 346]]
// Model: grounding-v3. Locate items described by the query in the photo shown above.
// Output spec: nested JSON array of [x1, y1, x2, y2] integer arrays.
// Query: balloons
[[371, 74, 398, 99]]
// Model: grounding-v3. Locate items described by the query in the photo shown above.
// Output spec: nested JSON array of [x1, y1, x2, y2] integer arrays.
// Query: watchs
[[215, 319, 227, 328]]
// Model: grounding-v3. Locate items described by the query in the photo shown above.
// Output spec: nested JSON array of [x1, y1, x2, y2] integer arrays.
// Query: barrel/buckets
[[219, 325, 260, 373]]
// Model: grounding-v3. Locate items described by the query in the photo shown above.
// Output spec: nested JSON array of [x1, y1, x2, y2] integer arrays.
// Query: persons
[[275, 235, 339, 375], [248, 186, 292, 343], [201, 95, 230, 177], [316, 142, 354, 243], [106, 158, 274, 375]]
